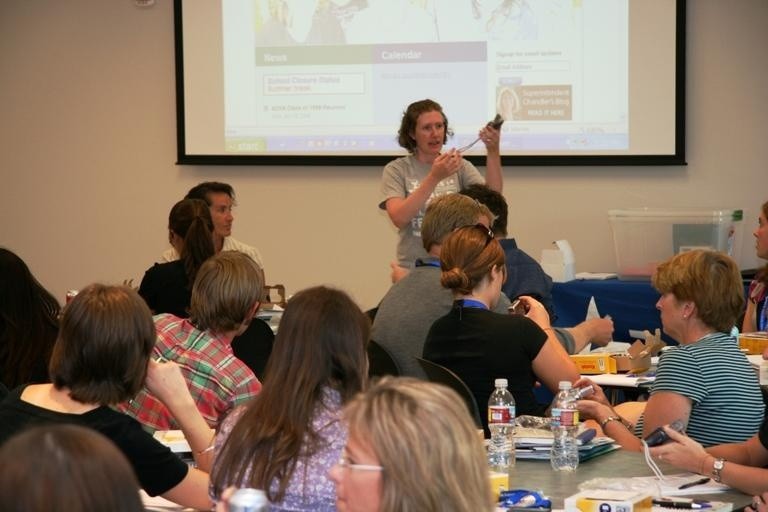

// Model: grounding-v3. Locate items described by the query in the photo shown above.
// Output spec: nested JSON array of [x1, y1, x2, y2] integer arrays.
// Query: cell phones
[[642, 419, 684, 446]]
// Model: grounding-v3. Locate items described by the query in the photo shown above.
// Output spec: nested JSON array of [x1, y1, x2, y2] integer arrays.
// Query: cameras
[[485, 113, 504, 130]]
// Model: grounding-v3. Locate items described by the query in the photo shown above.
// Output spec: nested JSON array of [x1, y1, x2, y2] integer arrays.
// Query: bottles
[[552, 380, 580, 472], [488, 378, 516, 469]]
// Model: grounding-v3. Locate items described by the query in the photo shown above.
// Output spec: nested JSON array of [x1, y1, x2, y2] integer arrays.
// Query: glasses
[[471, 223, 494, 265], [339, 448, 382, 473]]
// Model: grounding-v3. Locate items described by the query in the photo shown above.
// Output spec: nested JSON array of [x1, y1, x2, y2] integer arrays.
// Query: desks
[[484, 446, 758, 511], [534, 273, 752, 416]]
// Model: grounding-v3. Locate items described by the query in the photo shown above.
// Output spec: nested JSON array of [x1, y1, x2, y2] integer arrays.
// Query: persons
[[377, 100, 503, 271], [0, 181, 768, 512]]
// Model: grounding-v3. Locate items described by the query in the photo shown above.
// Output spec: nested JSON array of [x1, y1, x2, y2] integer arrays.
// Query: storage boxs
[[606, 206, 744, 281]]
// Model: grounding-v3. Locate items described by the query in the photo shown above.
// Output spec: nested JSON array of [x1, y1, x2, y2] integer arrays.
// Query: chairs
[[232, 316, 275, 381], [413, 356, 482, 430], [365, 337, 401, 376], [264, 284, 286, 307]]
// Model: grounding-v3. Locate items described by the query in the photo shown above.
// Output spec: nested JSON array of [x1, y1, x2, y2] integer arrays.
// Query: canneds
[[228, 488, 271, 512], [66, 290, 79, 303]]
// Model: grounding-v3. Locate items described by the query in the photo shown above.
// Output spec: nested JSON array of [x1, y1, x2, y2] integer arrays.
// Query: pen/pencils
[[678, 478, 710, 489], [652, 497, 712, 509]]
